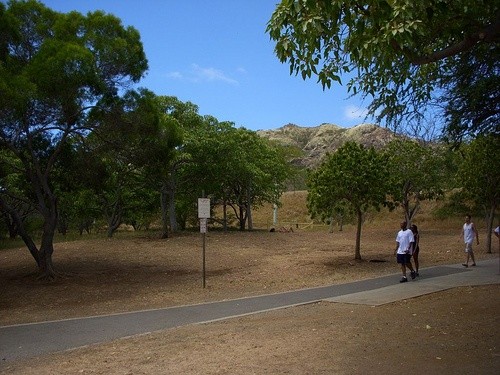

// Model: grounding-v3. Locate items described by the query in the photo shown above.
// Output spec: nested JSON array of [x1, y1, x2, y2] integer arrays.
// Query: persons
[[457, 214, 479, 268], [493, 227, 500, 236], [393, 221, 421, 283]]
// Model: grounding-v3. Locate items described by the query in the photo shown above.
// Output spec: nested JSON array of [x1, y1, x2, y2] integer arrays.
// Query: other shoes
[[462, 264, 468, 267], [415, 272, 419, 276], [400, 277, 408, 282], [471, 264, 475, 266], [411, 270, 416, 279]]
